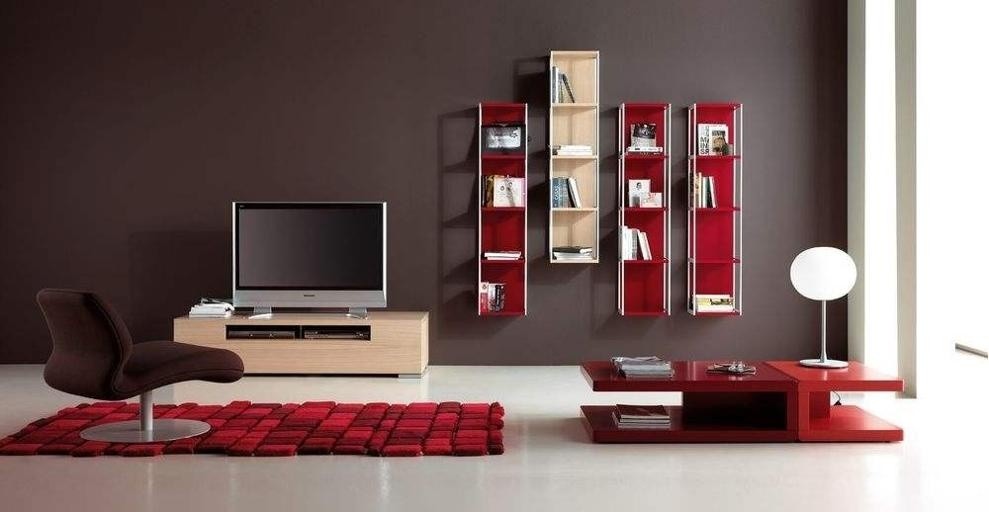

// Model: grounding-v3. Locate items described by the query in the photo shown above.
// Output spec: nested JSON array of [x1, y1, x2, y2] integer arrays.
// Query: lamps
[[789, 246, 858, 369]]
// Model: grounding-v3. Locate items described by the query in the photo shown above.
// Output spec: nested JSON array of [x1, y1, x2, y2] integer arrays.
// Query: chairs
[[37, 287, 244, 441]]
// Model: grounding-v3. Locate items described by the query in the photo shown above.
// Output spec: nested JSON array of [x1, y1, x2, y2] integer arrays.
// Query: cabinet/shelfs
[[173, 310, 429, 379]]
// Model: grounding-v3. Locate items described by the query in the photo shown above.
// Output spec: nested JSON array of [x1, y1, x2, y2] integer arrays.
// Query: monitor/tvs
[[231, 201, 388, 320]]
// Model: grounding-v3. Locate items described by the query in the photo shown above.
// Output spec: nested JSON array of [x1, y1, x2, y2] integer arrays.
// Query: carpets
[[0, 401, 505, 455]]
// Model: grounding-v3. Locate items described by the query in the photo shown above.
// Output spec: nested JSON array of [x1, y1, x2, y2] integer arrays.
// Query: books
[[552, 245, 594, 261], [552, 144, 593, 156], [188, 296, 235, 317], [553, 66, 576, 104], [483, 250, 522, 261], [612, 403, 671, 428], [552, 175, 582, 209], [691, 293, 735, 312], [619, 119, 729, 262], [612, 355, 674, 379]]
